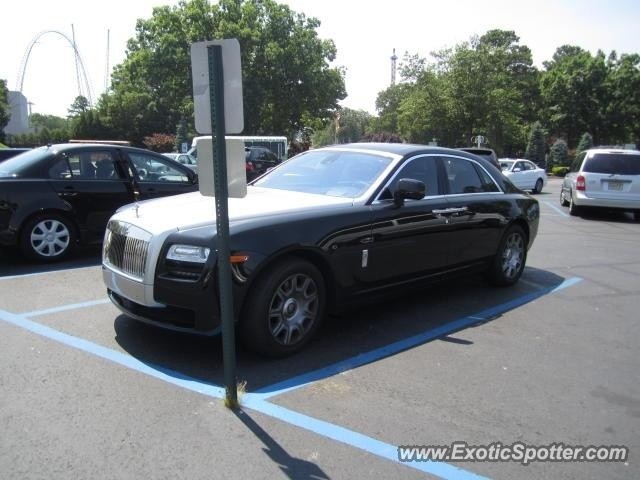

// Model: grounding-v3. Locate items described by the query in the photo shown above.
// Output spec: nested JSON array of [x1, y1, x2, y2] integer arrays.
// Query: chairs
[[96, 159, 130, 179]]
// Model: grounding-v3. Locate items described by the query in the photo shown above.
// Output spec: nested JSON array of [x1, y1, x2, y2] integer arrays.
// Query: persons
[[96, 159, 114, 176]]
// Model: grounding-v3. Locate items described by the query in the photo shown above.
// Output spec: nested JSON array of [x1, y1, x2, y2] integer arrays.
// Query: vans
[[559, 145, 640, 220]]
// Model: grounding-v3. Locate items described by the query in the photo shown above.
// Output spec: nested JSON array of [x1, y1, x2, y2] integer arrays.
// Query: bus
[[189, 135, 289, 158]]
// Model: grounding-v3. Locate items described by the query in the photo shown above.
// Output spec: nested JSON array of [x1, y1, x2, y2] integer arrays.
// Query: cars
[[88, 139, 546, 358]]
